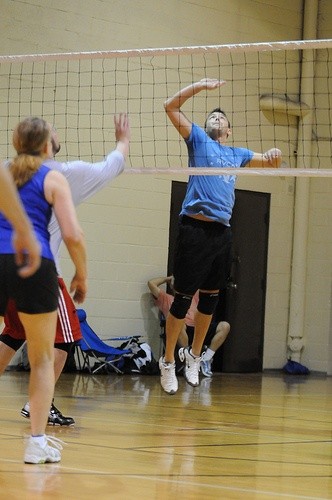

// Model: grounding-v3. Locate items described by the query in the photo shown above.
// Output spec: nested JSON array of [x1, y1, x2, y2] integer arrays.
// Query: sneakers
[[178, 346, 203, 386], [24, 436, 65, 465], [199, 352, 212, 377], [158, 355, 179, 393], [21, 397, 74, 427]]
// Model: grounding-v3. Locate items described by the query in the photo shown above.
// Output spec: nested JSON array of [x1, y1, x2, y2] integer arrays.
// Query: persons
[[160, 78, 282, 393], [0, 114, 130, 428], [0, 117, 87, 464], [148, 276, 231, 377]]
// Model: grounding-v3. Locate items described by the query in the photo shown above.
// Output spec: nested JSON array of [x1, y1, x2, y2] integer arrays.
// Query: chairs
[[158, 305, 186, 375], [76, 309, 143, 375]]
